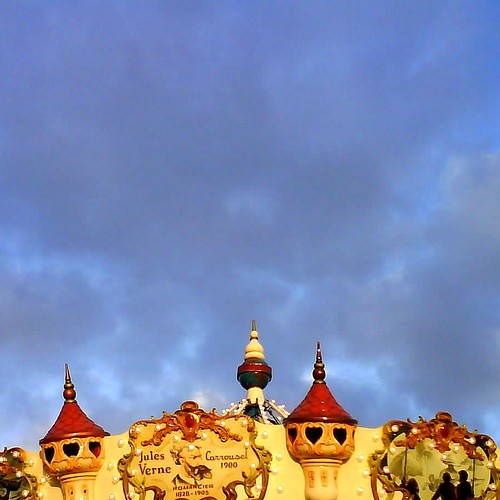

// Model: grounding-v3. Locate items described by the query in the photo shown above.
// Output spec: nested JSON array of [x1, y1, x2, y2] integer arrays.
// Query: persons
[[431, 472, 457, 500], [456, 470, 474, 500], [399, 477, 422, 500]]
